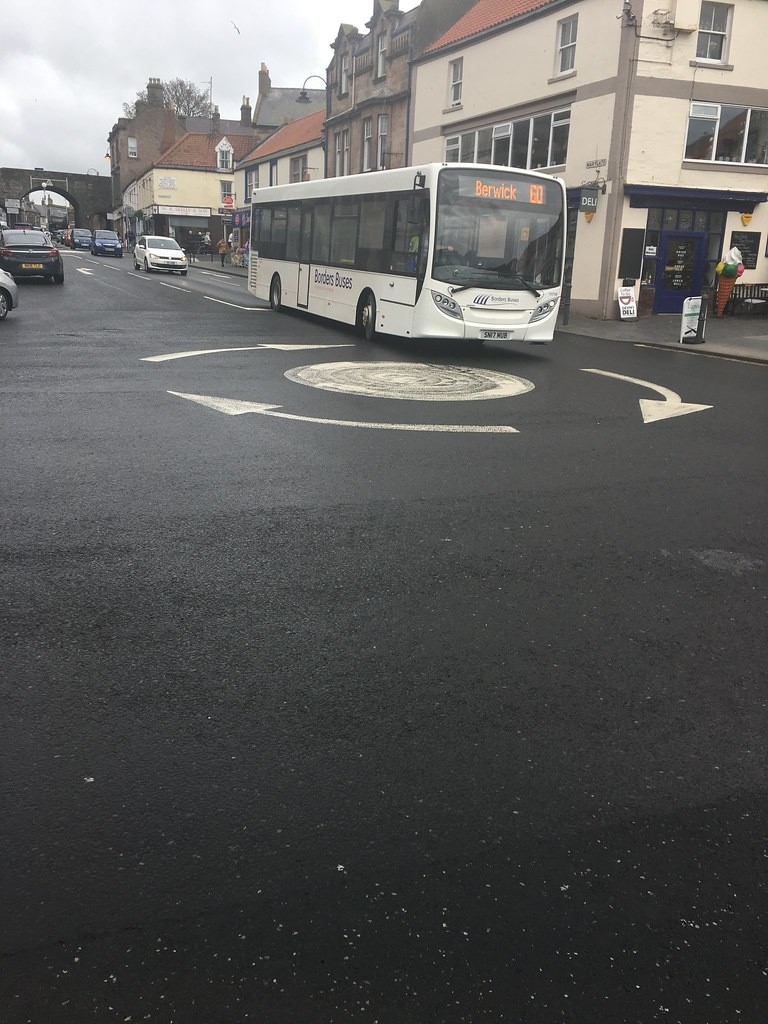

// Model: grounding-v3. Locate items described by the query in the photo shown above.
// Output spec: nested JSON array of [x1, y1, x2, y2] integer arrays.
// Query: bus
[[248, 163, 567, 344]]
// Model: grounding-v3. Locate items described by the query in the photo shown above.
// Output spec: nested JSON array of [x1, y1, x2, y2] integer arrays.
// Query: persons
[[188, 231, 212, 255], [228, 232, 233, 247], [407, 224, 453, 266], [217, 239, 228, 267]]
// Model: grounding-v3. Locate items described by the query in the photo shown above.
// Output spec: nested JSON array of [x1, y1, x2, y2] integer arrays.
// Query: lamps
[[104, 146, 120, 161], [296, 75, 328, 104]]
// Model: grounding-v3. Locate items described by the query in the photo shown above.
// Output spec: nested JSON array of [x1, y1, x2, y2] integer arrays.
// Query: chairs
[[75, 232, 79, 235], [740, 284, 766, 320]]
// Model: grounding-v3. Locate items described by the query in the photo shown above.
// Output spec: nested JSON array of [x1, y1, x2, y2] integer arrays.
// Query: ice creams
[[715, 246, 744, 316]]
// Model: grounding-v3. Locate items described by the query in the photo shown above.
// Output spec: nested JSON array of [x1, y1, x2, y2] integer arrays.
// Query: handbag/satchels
[[208, 242, 211, 246]]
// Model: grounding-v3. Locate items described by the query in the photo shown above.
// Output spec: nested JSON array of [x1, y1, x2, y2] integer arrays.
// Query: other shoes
[[207, 253, 211, 256], [221, 264, 224, 267]]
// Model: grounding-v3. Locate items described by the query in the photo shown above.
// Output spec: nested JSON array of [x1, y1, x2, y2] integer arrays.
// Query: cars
[[133, 234, 188, 276], [52, 228, 123, 259], [0, 221, 64, 319]]
